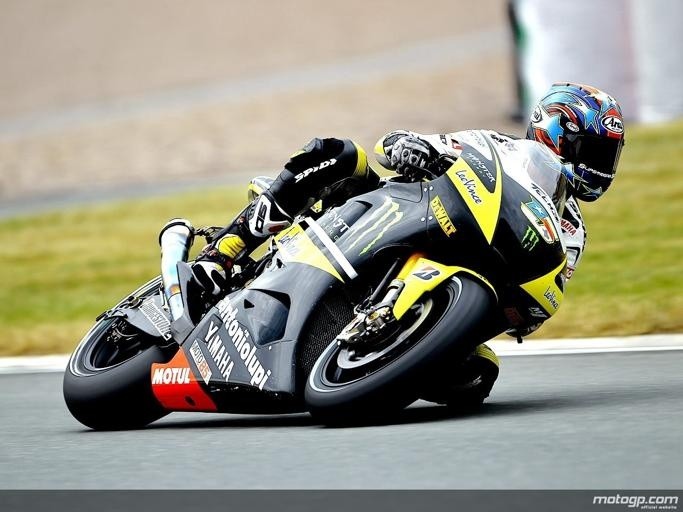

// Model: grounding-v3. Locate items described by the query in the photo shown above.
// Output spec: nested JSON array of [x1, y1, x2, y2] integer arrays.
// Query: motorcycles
[[62, 129, 572, 433]]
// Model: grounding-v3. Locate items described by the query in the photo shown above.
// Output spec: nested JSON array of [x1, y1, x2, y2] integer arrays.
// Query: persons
[[190, 81, 625, 409]]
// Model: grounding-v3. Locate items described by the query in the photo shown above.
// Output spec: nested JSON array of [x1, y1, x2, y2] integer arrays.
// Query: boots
[[192, 191, 292, 292]]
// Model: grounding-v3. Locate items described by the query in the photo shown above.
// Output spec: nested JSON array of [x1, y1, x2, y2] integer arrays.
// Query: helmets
[[527, 84, 627, 203]]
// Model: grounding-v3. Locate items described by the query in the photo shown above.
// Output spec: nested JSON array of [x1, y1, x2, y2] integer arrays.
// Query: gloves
[[388, 134, 432, 180]]
